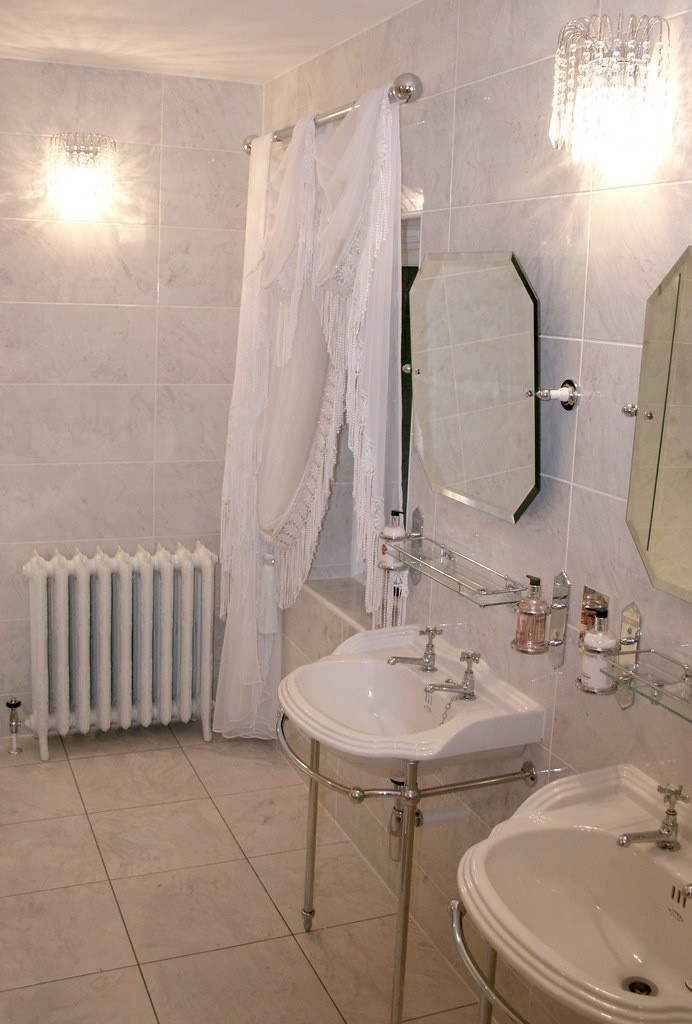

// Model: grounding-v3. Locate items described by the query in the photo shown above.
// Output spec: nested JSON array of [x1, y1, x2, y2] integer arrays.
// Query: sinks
[[457, 762, 692, 1024], [278, 624, 542, 760]]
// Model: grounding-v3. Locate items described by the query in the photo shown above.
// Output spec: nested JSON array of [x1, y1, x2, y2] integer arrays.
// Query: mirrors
[[408, 246, 542, 523], [622, 245, 691, 605]]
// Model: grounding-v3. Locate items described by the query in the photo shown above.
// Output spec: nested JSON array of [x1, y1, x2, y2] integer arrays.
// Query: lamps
[[45, 131, 119, 226], [549, 14, 680, 184]]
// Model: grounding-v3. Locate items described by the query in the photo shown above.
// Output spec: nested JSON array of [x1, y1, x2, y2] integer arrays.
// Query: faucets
[[616, 781, 690, 850], [387, 626, 443, 671], [424, 652, 480, 698]]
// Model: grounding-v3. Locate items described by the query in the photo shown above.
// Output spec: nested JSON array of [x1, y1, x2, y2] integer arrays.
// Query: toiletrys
[[576, 602, 617, 690], [514, 575, 549, 654], [383, 505, 411, 567]]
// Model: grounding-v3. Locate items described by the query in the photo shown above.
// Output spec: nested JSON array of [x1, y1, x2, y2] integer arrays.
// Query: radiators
[[20, 540, 218, 763]]
